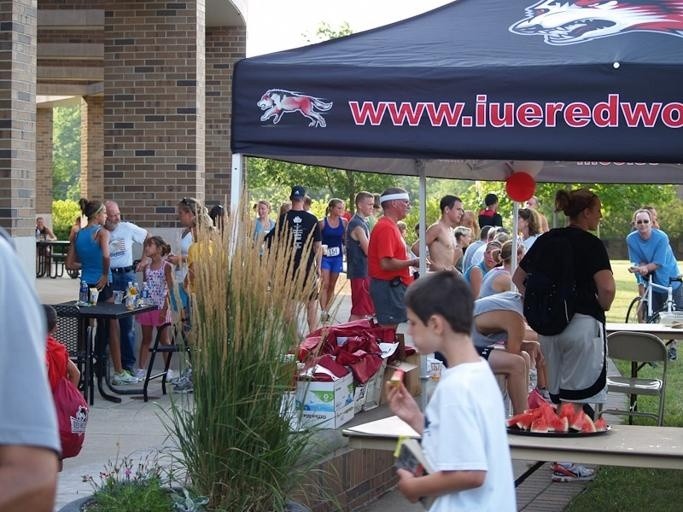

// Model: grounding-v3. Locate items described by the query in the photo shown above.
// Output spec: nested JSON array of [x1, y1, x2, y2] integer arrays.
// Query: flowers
[[74, 443, 185, 511]]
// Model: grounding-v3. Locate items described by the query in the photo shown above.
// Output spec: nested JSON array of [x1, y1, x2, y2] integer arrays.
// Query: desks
[[602, 323, 682, 411], [31, 291, 160, 403], [339, 413, 682, 496]]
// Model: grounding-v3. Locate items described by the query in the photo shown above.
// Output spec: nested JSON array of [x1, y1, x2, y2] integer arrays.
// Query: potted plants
[[55, 183, 349, 512]]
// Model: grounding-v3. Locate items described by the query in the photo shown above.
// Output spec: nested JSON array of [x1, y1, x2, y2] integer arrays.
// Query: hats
[[291, 185, 306, 197]]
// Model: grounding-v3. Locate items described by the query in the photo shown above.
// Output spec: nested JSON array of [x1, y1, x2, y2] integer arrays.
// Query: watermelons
[[506, 402, 608, 434], [389, 368, 404, 388]]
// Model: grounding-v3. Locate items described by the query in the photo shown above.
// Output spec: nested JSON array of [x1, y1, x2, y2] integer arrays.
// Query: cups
[[112, 290, 122, 304], [430, 360, 442, 381]]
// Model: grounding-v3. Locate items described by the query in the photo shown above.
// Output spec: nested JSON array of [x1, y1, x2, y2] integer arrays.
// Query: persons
[[513, 187, 616, 482], [35, 218, 57, 241], [252, 187, 549, 424], [384, 270, 517, 512], [65, 199, 228, 395], [0, 227, 64, 512], [626, 206, 683, 360], [41, 305, 80, 390]]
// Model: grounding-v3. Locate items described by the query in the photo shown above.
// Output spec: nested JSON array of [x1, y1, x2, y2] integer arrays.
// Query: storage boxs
[[354, 343, 423, 414], [294, 371, 354, 431]]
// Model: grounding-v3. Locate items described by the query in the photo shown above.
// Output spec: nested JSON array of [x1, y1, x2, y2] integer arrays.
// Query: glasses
[[637, 220, 649, 224], [181, 196, 195, 216]]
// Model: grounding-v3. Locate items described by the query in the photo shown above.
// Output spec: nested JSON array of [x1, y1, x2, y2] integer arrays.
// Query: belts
[[111, 266, 130, 273]]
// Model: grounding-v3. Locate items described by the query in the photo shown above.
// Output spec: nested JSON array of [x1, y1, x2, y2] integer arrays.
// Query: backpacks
[[47, 346, 90, 458], [522, 230, 597, 337]]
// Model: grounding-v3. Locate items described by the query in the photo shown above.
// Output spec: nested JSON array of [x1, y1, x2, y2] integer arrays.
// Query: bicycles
[[625, 268, 682, 323]]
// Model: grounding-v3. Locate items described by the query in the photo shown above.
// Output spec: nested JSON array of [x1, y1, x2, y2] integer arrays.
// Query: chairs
[[143, 296, 194, 400], [36, 239, 81, 278], [595, 328, 668, 427], [47, 302, 96, 408]]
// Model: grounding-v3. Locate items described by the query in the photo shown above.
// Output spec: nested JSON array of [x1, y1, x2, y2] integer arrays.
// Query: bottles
[[125, 281, 148, 310], [78, 280, 88, 301], [35, 229, 40, 242]]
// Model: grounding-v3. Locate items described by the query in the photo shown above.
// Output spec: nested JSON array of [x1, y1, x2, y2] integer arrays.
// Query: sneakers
[[551, 461, 597, 483], [668, 339, 679, 361], [111, 367, 146, 386], [168, 367, 195, 394]]
